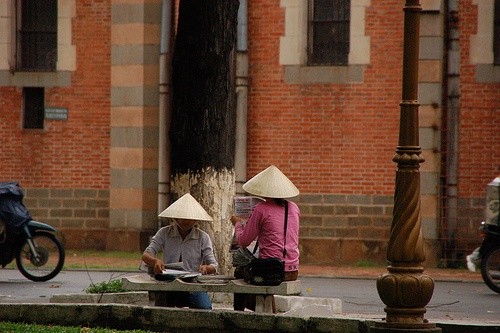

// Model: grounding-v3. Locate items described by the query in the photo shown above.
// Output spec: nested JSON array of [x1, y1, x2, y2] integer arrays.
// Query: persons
[[231, 164, 301, 310], [142, 192, 220, 310]]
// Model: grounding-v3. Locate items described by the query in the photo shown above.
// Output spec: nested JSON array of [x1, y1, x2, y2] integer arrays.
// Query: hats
[[156, 193, 214, 222], [243, 164, 300, 199]]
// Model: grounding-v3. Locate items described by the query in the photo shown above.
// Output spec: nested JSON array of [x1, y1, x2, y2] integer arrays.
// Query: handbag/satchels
[[248, 258, 285, 286]]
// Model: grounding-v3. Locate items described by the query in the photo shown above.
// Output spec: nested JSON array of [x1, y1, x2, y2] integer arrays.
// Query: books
[[144, 262, 202, 280]]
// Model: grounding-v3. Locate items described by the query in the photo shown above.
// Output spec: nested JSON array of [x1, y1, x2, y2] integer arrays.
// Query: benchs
[[122, 272, 302, 314]]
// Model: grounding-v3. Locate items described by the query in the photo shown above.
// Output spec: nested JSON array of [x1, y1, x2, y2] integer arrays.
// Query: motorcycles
[[0, 180, 65, 282], [465, 222, 500, 296]]
